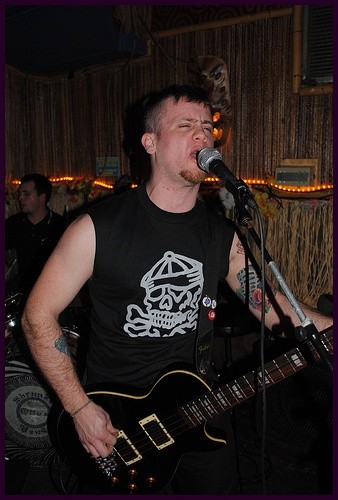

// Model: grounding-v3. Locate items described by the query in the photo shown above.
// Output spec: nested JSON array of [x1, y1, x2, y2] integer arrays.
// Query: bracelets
[[69, 398, 92, 417]]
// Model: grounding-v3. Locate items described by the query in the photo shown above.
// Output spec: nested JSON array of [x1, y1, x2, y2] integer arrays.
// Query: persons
[[1, 173, 67, 287], [19, 83, 334, 495]]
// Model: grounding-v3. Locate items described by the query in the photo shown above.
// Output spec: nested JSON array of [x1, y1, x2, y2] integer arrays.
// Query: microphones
[[198, 148, 259, 211]]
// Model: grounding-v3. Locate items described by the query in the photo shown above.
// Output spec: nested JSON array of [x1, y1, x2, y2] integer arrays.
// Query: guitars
[[56, 325, 333, 497]]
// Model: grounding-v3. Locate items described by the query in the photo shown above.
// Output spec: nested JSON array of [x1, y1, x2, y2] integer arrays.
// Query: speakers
[[254, 350, 333, 474]]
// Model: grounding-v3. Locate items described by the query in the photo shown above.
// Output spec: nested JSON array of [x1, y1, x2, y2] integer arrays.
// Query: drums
[[6, 329, 89, 449]]
